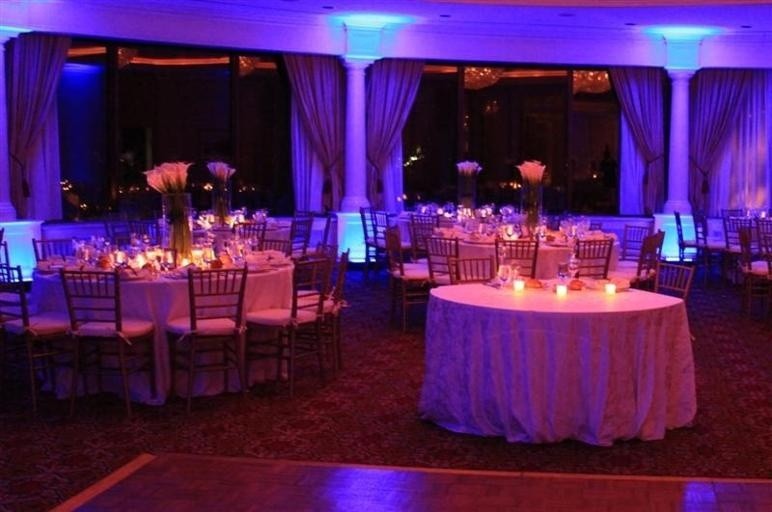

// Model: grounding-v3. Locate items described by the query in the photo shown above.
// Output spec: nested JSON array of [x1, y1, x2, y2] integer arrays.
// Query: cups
[[513, 279, 524, 295], [556, 287, 568, 300], [604, 284, 616, 300]]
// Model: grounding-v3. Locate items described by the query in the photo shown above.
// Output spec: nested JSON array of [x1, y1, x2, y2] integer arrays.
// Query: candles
[[556, 284, 567, 300], [513, 280, 525, 295], [603, 284, 616, 298]]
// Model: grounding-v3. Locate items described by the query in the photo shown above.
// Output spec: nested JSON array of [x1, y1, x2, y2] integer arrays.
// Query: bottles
[[744, 207, 768, 221]]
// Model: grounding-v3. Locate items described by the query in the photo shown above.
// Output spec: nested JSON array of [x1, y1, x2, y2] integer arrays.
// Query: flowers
[[456, 160, 482, 176], [208, 161, 236, 182], [514, 159, 547, 183], [145, 162, 196, 194]]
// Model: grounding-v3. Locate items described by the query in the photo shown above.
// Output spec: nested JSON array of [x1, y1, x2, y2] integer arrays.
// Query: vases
[[215, 184, 231, 224], [521, 183, 540, 226], [462, 176, 477, 209], [162, 194, 192, 258]]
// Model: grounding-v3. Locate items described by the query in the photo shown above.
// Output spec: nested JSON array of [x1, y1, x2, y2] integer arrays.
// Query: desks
[[421, 279, 697, 449]]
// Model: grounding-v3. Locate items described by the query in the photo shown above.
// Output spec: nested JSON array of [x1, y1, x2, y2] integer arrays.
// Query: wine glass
[[34, 231, 285, 279], [420, 203, 614, 246], [201, 209, 266, 228], [555, 258, 581, 285], [495, 246, 522, 292]]
